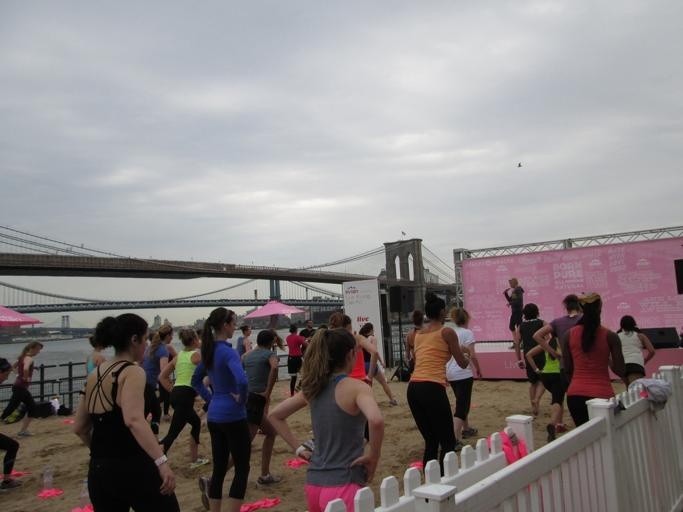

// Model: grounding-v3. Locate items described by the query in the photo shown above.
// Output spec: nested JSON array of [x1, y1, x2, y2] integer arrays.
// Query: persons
[[0, 358, 19, 488], [516, 294, 655, 443], [0, 341, 42, 436], [84, 335, 105, 381], [74, 313, 180, 511], [144, 293, 481, 512], [504, 279, 523, 350]]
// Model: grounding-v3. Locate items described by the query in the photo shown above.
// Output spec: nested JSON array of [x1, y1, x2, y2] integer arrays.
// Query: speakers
[[673, 259, 683, 295], [389, 285, 415, 312], [639, 327, 679, 348]]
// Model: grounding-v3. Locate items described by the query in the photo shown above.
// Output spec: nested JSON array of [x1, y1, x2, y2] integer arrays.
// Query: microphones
[[503, 288, 511, 295]]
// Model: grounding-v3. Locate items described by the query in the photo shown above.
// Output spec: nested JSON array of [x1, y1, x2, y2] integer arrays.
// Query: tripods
[[390, 313, 414, 381]]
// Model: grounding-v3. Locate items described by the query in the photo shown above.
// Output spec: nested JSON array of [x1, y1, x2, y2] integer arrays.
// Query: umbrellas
[[0, 304, 44, 328]]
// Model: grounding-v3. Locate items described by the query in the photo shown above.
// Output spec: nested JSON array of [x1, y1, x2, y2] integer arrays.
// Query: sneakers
[[189, 459, 213, 510], [17, 432, 36, 437], [546, 422, 568, 443], [0, 480, 23, 489], [456, 427, 478, 451], [255, 472, 282, 486]]
